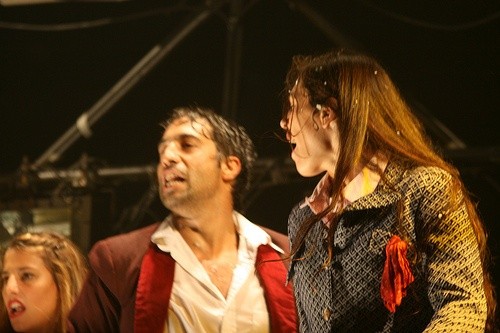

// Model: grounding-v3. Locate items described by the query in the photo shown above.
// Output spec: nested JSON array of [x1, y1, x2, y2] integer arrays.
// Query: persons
[[285, 47, 497, 333], [0, 226, 87, 333], [68, 104, 299, 333]]
